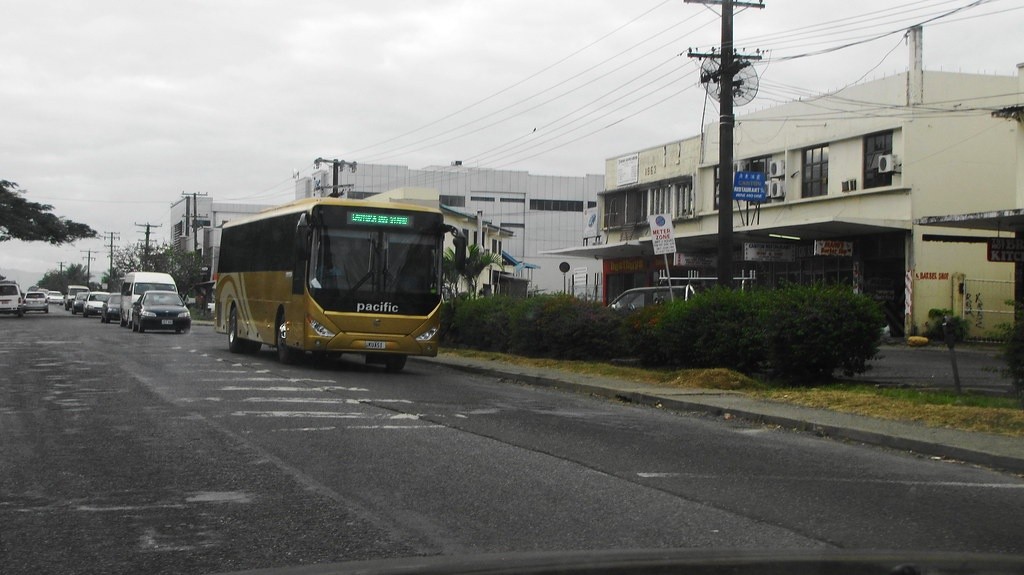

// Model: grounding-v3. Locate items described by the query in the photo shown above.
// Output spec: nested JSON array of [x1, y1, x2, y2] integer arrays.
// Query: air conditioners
[[766, 181, 772, 197], [771, 181, 785, 198], [770, 160, 785, 178], [879, 154, 899, 173]]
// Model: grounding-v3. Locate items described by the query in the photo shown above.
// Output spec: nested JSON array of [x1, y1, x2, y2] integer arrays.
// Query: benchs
[[583, 235, 601, 246]]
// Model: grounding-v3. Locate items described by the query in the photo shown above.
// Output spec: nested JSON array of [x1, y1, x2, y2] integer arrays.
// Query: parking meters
[[941, 323, 962, 394]]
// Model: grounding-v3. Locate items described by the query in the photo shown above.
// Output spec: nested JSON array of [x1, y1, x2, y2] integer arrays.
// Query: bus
[[214, 196, 466, 372]]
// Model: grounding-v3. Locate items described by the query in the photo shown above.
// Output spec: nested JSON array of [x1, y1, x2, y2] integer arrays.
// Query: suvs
[[0, 284, 24, 318]]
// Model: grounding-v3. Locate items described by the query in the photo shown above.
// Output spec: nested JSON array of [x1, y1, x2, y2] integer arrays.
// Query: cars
[[100, 293, 122, 324], [71, 292, 89, 315], [131, 289, 192, 334], [47, 291, 64, 305], [605, 286, 692, 311], [22, 292, 50, 313], [83, 291, 109, 317]]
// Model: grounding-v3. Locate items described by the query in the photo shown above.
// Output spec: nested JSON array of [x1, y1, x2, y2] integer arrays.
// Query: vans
[[64, 285, 90, 311], [120, 273, 179, 329]]
